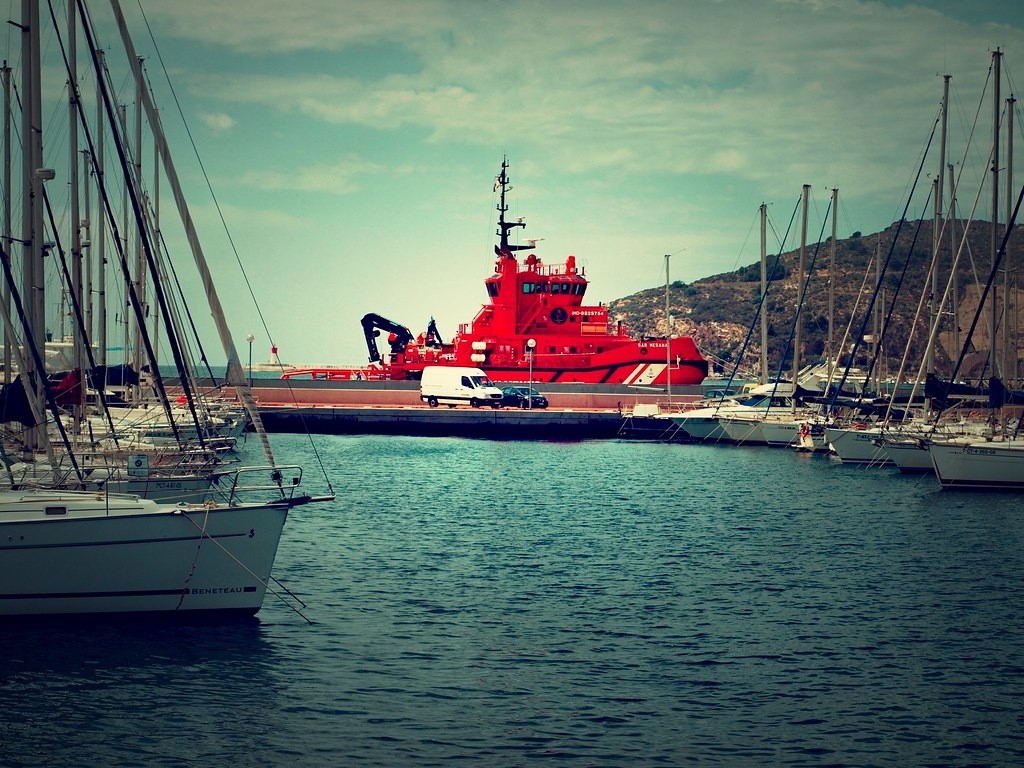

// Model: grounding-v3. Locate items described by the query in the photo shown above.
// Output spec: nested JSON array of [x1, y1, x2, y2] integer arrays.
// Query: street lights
[[246, 334, 255, 387], [527, 338, 538, 409]]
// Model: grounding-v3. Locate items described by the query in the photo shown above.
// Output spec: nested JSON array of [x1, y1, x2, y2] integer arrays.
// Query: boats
[[277, 153, 710, 387]]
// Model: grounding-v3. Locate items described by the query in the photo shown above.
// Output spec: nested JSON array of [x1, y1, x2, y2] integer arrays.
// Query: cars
[[499, 385, 549, 409]]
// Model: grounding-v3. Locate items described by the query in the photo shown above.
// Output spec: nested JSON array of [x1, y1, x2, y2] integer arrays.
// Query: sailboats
[[1, 1, 337, 624], [671, 45, 1023, 489]]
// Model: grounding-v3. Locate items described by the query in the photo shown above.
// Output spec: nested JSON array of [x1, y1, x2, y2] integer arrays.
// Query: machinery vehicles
[[360, 312, 452, 379]]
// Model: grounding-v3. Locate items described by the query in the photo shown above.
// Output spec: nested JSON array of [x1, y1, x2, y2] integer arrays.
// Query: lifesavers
[[854, 424, 866, 430], [800, 425, 809, 438]]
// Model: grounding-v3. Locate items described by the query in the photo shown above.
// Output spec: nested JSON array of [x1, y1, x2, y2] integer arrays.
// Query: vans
[[419, 365, 504, 409]]
